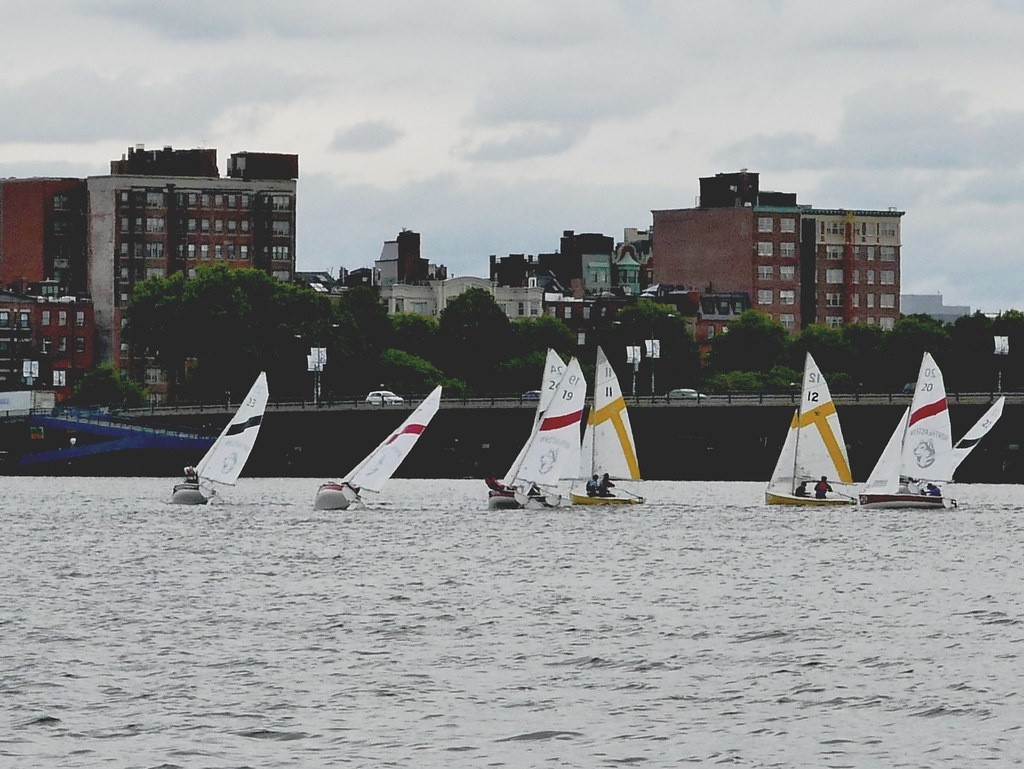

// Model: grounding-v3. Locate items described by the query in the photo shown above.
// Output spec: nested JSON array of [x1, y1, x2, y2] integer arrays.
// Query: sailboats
[[896, 395, 1007, 500], [312, 384, 444, 511], [487, 352, 588, 509], [859, 352, 959, 509], [567, 346, 645, 505], [764, 351, 862, 508], [170, 370, 270, 507], [483, 346, 567, 492]]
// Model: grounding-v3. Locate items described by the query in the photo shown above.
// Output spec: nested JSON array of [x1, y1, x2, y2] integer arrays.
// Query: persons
[[586, 474, 616, 499], [184, 463, 199, 484], [925, 483, 942, 497], [814, 476, 832, 499], [794, 481, 811, 498]]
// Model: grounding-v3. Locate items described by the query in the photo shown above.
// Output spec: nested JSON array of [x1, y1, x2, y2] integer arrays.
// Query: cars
[[663, 388, 708, 401], [522, 390, 542, 398], [902, 383, 918, 395], [365, 390, 403, 407]]
